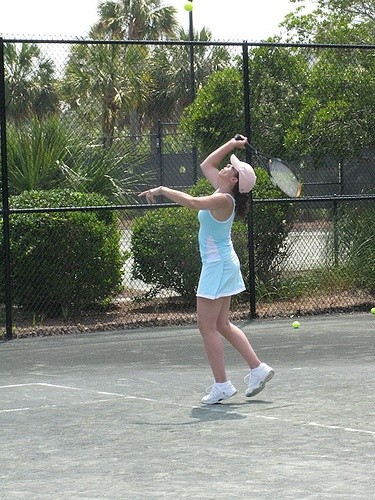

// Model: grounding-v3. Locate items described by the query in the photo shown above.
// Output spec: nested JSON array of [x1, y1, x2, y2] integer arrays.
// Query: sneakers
[[244, 363, 275, 397], [202, 380, 237, 405]]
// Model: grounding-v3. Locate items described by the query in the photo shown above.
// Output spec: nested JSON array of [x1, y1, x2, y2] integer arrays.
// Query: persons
[[138, 134, 274, 407]]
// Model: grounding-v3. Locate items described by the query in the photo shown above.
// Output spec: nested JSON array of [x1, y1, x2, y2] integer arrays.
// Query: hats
[[230, 153, 257, 194]]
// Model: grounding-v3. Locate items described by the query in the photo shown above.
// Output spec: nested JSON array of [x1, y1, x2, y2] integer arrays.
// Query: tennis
[[370, 307, 375, 315], [183, 1, 193, 12], [292, 321, 301, 329]]
